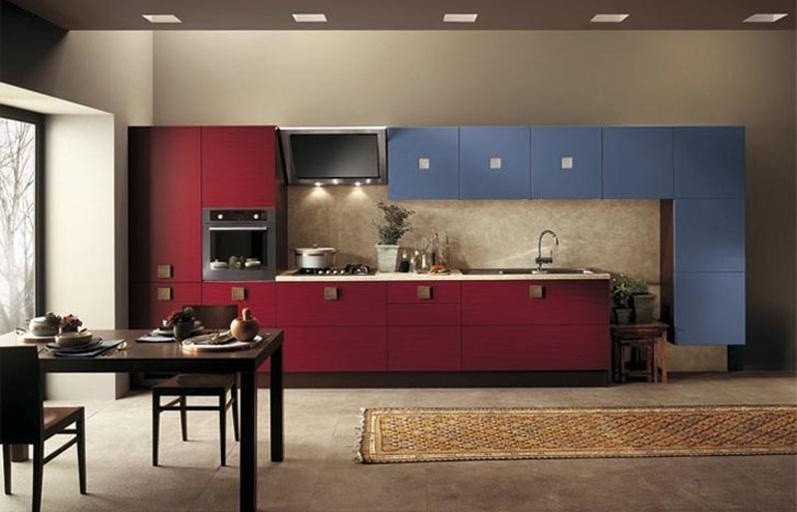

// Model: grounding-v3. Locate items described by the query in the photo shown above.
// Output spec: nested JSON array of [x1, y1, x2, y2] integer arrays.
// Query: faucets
[[536, 230, 559, 272]]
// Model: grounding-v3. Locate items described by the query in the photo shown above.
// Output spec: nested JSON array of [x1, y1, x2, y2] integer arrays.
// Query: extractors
[[277, 126, 387, 188]]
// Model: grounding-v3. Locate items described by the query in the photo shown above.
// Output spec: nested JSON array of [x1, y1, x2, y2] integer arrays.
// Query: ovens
[[200, 206, 287, 282]]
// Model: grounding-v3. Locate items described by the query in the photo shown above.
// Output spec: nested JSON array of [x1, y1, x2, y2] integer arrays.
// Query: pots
[[294, 244, 340, 269]]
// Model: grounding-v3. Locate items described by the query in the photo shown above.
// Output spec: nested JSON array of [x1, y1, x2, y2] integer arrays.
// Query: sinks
[[458, 268, 599, 275]]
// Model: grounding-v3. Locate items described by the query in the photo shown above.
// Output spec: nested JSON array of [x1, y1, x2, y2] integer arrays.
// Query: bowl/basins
[[54, 332, 92, 345], [28, 316, 59, 336]]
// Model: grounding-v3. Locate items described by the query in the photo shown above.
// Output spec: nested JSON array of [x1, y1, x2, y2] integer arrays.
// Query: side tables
[[612, 321, 670, 385]]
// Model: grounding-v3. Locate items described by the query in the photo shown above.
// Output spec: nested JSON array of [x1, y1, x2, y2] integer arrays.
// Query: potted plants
[[612, 275, 655, 324]]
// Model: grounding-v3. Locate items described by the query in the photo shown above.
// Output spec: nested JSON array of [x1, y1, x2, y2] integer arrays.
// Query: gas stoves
[[293, 263, 377, 276]]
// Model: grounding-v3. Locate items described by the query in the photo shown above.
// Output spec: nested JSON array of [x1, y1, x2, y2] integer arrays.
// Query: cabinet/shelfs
[[461, 281, 617, 387], [388, 284, 461, 387], [388, 127, 532, 198], [660, 198, 746, 274], [130, 283, 273, 388], [273, 281, 388, 388], [674, 125, 746, 199], [127, 125, 276, 282], [531, 128, 674, 201], [661, 274, 747, 345]]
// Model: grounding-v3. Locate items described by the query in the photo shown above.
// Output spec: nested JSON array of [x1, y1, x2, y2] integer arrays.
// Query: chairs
[[2, 346, 88, 511], [149, 302, 240, 465]]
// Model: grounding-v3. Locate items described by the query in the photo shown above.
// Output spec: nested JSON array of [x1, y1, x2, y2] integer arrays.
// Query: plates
[[181, 333, 262, 349], [43, 336, 103, 352], [152, 326, 204, 335]]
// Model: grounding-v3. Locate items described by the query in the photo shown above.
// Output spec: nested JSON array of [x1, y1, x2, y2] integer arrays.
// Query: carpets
[[357, 401, 795, 464]]
